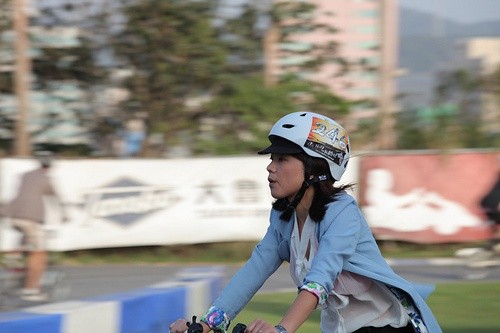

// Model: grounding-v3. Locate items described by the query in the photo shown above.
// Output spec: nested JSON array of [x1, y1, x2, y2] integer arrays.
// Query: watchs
[[274, 325, 288, 333]]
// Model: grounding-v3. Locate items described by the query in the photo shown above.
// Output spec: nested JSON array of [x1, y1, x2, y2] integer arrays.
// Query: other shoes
[[19, 287, 43, 300]]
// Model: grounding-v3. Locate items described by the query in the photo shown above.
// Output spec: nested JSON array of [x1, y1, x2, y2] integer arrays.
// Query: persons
[[168, 111, 443, 333], [0, 157, 70, 302]]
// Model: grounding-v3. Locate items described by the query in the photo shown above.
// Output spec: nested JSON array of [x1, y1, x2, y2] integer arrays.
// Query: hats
[[39, 156, 52, 167], [259, 110, 350, 185]]
